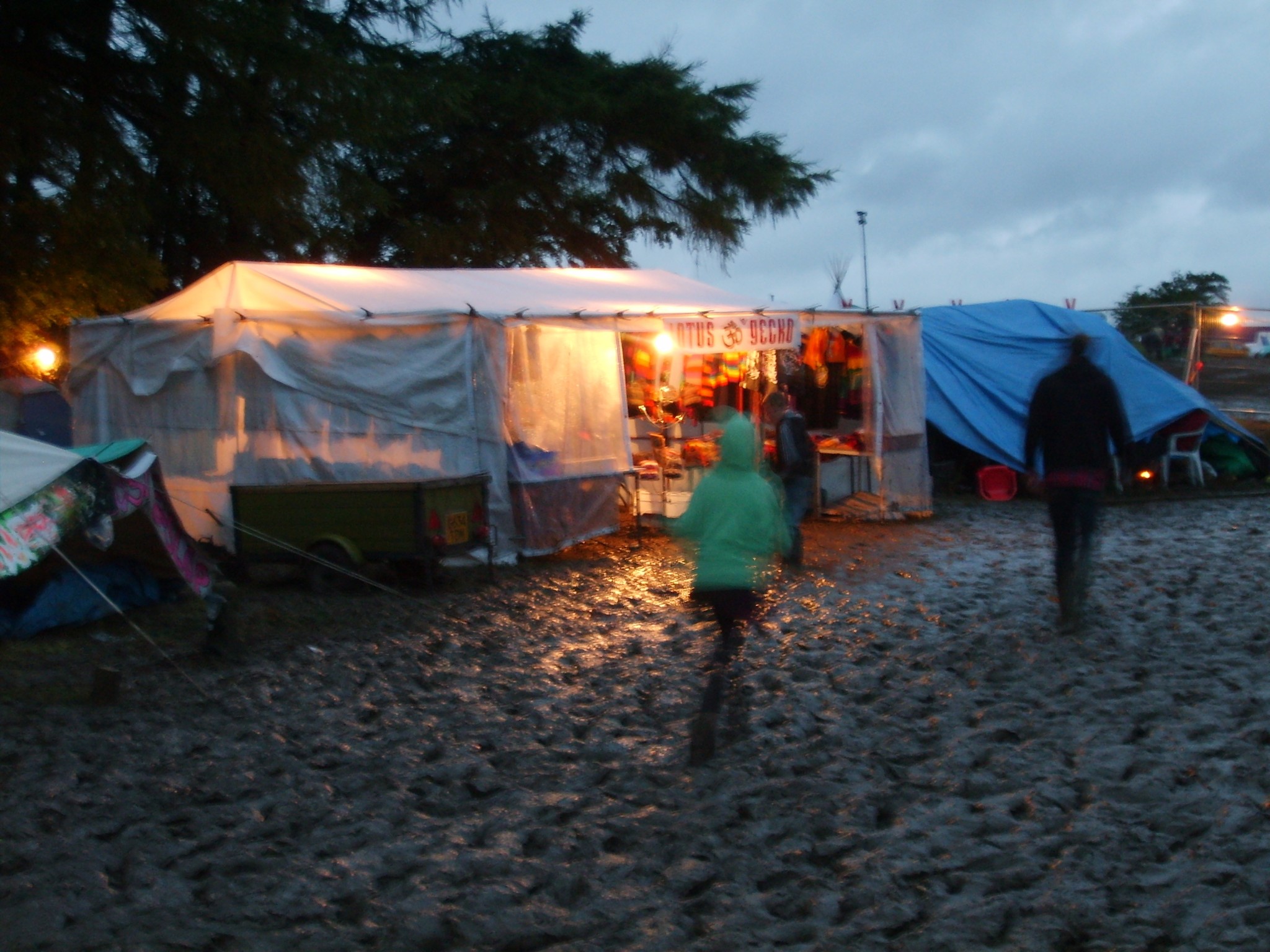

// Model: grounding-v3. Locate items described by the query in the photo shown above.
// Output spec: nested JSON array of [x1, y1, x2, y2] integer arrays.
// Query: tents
[[0, 260, 931, 704], [915, 299, 1270, 482]]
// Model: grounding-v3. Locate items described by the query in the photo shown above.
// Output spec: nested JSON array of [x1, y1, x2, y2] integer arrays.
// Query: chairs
[[1152, 411, 1210, 488]]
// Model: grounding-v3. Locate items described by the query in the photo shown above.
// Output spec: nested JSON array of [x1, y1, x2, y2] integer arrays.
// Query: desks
[[514, 442, 872, 552]]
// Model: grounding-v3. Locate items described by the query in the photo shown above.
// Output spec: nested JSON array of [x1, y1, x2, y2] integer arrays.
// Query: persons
[[1023, 331, 1141, 635], [762, 390, 819, 582], [660, 411, 797, 758]]
